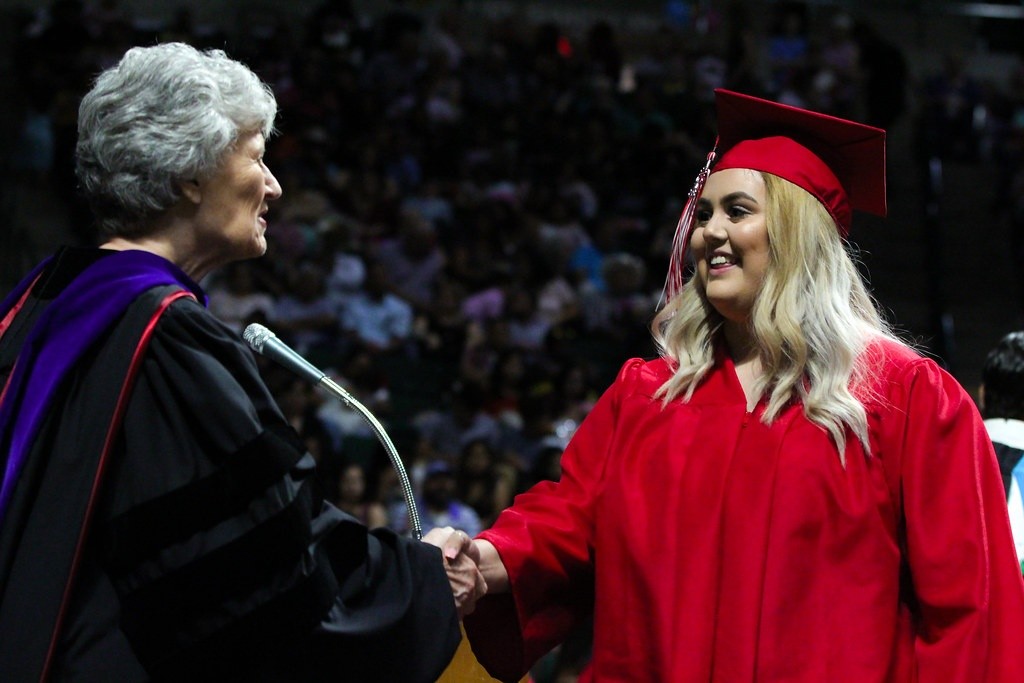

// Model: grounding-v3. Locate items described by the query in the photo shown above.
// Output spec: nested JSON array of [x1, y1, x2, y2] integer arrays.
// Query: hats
[[654, 87, 888, 311]]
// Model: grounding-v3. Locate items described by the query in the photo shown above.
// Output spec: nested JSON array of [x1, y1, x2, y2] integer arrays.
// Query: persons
[[419, 85, 1024, 683], [0, 41, 491, 682], [0, 1, 1023, 536], [979, 329, 1024, 578]]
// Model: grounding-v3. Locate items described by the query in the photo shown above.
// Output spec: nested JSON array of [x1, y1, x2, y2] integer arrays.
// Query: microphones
[[244, 323, 423, 542]]
